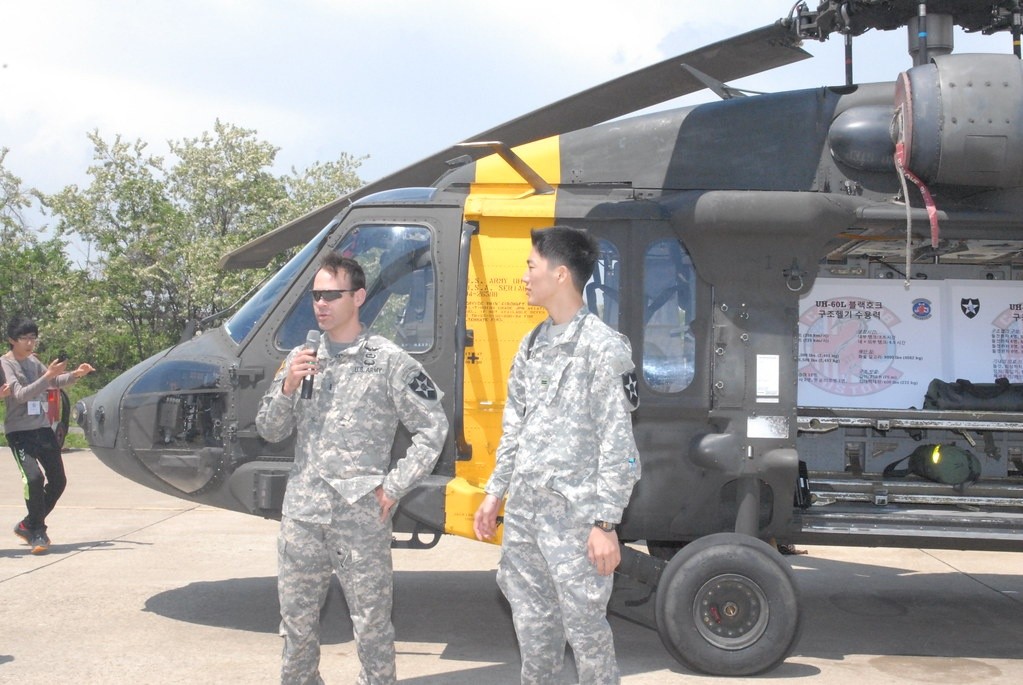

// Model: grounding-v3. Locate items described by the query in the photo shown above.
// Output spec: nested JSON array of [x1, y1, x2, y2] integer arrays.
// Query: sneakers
[[14, 522, 51, 553]]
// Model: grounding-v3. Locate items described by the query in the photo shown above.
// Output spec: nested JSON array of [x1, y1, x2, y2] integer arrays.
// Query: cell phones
[[58, 353, 68, 364]]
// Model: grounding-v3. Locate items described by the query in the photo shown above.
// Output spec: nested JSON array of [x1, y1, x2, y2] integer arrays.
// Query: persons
[[474, 225, 643, 685], [255, 251, 449, 685], [0, 309, 96, 555]]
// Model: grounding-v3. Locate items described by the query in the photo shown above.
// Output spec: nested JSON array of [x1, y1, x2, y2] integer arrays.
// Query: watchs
[[594, 520, 617, 532]]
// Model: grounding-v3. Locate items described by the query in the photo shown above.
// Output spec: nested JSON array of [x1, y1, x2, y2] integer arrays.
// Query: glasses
[[310, 290, 355, 302], [17, 336, 36, 343]]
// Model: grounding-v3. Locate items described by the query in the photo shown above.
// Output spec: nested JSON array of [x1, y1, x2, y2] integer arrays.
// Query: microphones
[[301, 330, 321, 399]]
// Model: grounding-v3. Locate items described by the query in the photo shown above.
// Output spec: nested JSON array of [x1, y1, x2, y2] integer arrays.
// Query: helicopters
[[72, 0, 1023, 676]]
